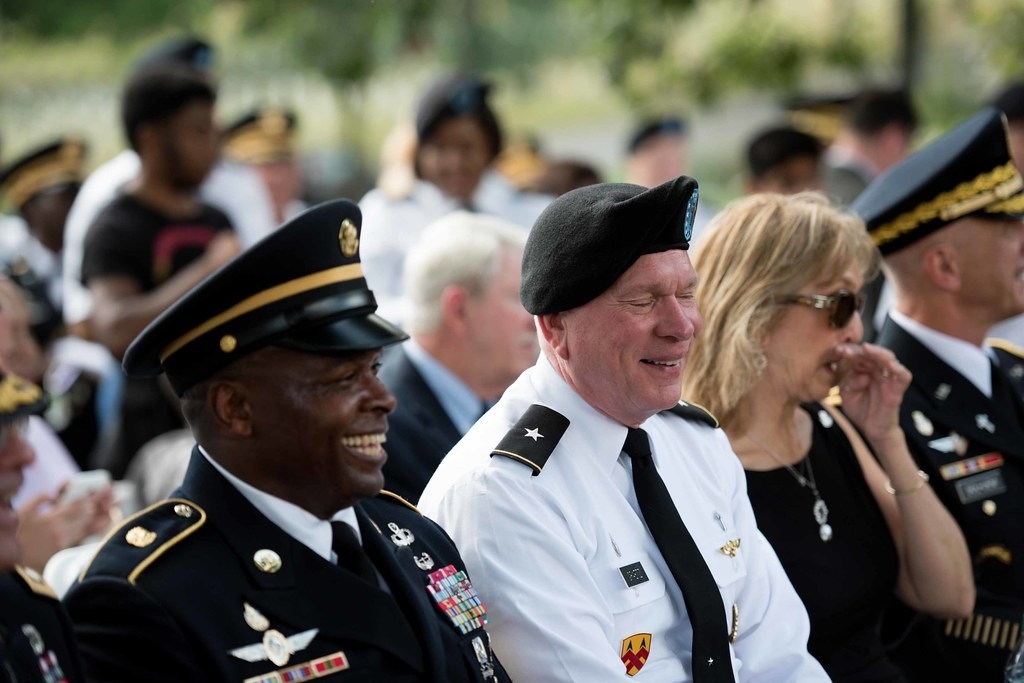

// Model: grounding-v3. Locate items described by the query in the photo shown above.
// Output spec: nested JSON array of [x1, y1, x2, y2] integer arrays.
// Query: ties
[[990, 361, 1022, 432], [332, 522, 379, 589], [623, 423, 736, 682]]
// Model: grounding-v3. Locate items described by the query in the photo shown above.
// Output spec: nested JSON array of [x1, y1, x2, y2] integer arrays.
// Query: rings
[[882, 371, 889, 378]]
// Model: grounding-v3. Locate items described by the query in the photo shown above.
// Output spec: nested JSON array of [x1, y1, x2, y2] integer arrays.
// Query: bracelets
[[884, 471, 929, 495]]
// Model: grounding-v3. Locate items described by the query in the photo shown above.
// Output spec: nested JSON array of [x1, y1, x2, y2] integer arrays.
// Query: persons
[[0, 34, 1024, 598], [681, 190, 977, 683], [375, 210, 541, 507], [0, 367, 63, 683], [414, 175, 832, 683], [61, 198, 511, 683], [847, 106, 1024, 683]]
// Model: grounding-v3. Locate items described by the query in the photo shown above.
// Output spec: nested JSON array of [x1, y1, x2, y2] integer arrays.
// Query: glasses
[[769, 293, 870, 329]]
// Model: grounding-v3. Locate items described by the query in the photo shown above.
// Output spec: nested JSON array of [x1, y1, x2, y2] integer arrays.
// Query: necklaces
[[743, 432, 831, 541]]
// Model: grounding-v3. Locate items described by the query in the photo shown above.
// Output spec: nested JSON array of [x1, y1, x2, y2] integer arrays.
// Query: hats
[[123, 200, 410, 398], [0, 139, 86, 215], [0, 366, 52, 422], [219, 113, 300, 166], [852, 110, 1024, 260], [518, 177, 701, 316]]
